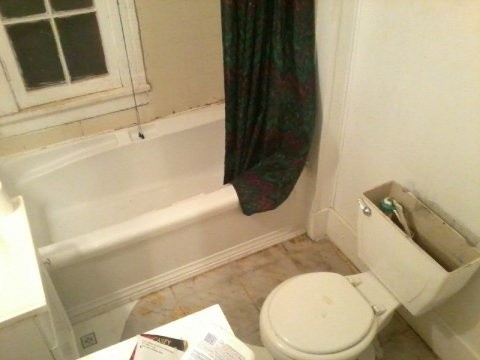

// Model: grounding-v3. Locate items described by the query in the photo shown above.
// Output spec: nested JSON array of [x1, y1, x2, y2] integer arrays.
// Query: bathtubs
[[0, 99, 312, 325]]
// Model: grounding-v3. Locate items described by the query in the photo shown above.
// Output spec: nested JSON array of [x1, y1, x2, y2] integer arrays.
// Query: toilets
[[259, 179, 479, 360]]
